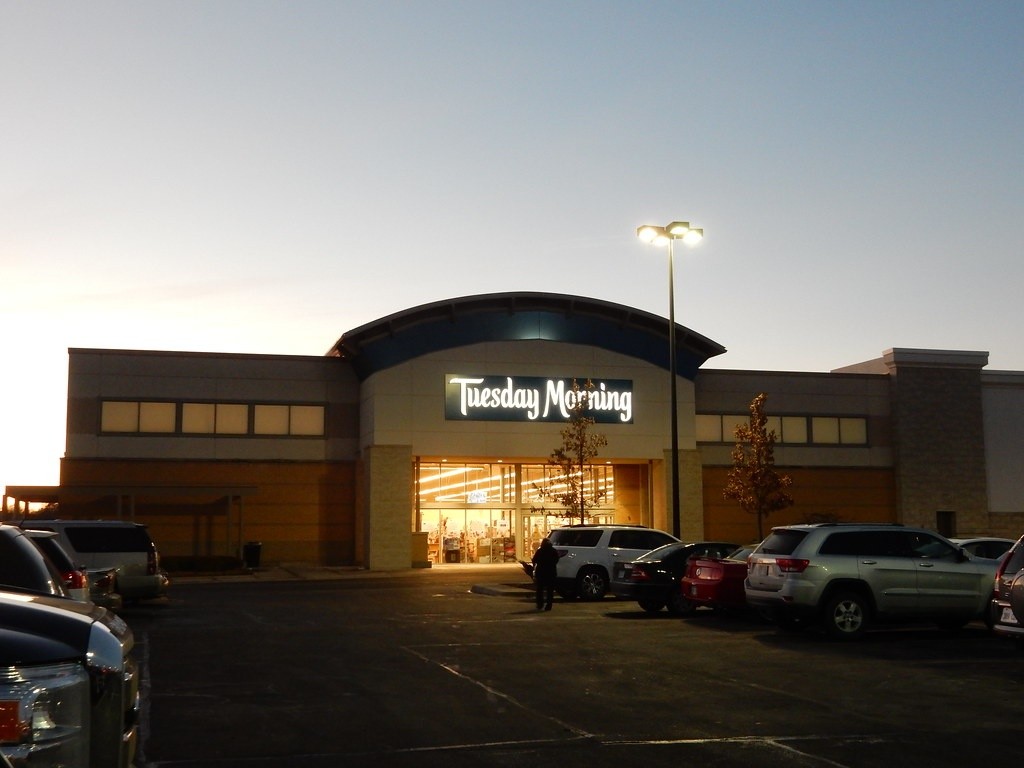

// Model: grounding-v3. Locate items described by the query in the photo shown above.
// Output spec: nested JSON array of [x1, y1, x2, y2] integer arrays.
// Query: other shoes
[[545, 602, 552, 611]]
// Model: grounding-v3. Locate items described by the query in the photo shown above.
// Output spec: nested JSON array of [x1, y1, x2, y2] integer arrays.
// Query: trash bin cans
[[936, 510, 957, 539], [243, 540, 263, 570]]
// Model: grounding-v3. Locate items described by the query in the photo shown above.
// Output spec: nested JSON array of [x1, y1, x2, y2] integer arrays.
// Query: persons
[[533, 538, 559, 611]]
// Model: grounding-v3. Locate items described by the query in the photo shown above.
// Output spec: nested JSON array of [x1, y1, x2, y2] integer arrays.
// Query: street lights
[[636, 222, 704, 541]]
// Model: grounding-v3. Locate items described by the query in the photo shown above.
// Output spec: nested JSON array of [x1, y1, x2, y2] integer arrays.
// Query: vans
[[1, 520, 169, 605]]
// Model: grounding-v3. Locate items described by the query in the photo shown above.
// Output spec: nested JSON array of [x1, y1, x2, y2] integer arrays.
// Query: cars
[[948, 537, 1017, 561], [682, 543, 761, 608], [0, 585, 143, 768], [992, 534, 1024, 644], [0, 524, 122, 611], [744, 521, 1001, 641], [611, 541, 742, 616], [533, 525, 684, 602]]
